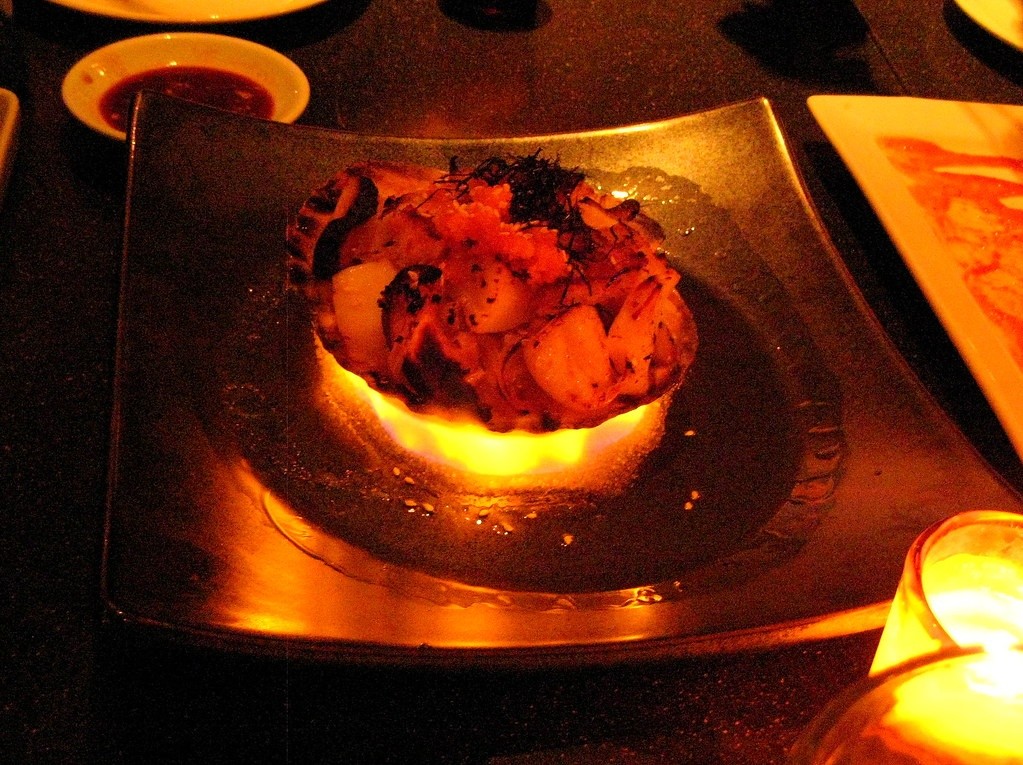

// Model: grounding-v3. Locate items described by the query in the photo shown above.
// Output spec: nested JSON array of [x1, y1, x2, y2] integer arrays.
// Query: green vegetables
[[435, 146, 599, 302]]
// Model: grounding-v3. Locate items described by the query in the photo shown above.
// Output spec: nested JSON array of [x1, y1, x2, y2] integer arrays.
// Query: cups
[[786, 646, 1023, 765], [869, 510, 1023, 675]]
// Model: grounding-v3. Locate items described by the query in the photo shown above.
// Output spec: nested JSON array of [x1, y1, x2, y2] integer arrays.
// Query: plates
[[62, 30, 311, 145], [102, 85, 1023, 670], [954, 0, 1023, 52], [0, 87, 22, 196], [48, 0, 331, 25]]
